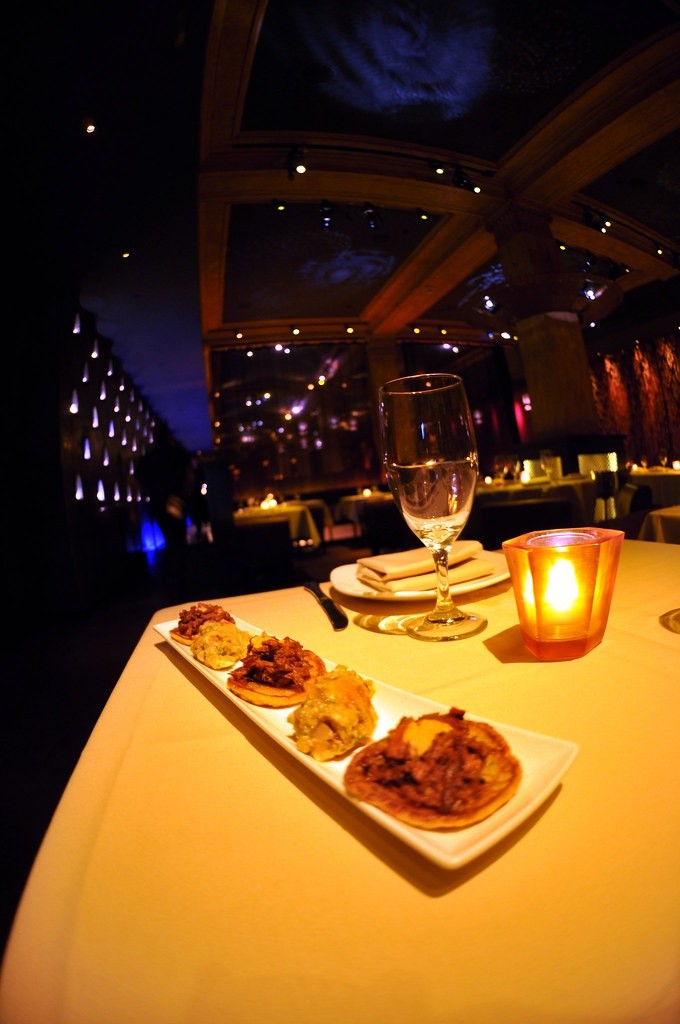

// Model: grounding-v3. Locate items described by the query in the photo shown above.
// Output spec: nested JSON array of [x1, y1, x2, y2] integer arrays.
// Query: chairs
[[616, 483, 653, 519], [523, 457, 564, 477], [364, 501, 425, 555], [478, 498, 575, 552], [579, 452, 619, 475]]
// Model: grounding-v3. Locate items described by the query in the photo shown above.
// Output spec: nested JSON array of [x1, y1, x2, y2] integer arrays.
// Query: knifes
[[303, 583, 349, 631]]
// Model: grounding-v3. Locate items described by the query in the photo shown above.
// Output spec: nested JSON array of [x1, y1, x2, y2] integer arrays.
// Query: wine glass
[[658, 450, 667, 466], [378, 373, 488, 642]]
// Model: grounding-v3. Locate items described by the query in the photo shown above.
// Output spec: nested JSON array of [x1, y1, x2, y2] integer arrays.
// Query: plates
[[155, 614, 578, 869], [330, 552, 511, 601]]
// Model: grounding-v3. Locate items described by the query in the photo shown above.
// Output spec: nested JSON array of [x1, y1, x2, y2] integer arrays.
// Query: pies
[[227, 650, 327, 707], [170, 618, 235, 645], [345, 712, 522, 830]]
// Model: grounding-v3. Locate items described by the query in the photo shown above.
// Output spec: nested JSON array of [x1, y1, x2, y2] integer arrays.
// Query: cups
[[503, 527, 625, 660], [545, 458, 563, 480]]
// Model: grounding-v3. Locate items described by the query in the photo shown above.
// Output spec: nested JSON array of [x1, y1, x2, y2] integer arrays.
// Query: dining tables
[[0, 538, 680, 1024], [233, 467, 680, 544]]
